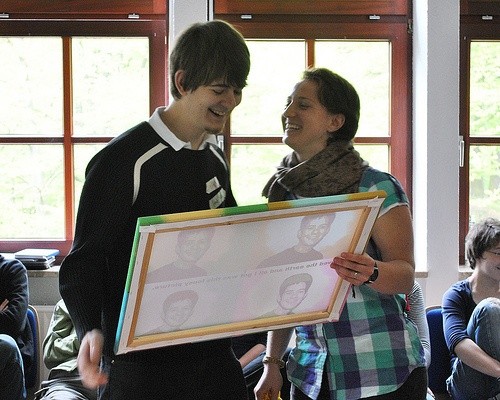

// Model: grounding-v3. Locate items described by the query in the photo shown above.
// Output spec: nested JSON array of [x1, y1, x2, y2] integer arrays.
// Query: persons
[[407, 281, 432, 369], [441, 218, 500, 400], [39, 299, 97, 400], [58, 20, 250, 400], [232, 332, 268, 376], [0, 254, 29, 400], [254, 68, 428, 400]]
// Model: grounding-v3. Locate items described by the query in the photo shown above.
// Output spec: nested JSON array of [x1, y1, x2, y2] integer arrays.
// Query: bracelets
[[364, 263, 378, 285], [262, 356, 285, 367]]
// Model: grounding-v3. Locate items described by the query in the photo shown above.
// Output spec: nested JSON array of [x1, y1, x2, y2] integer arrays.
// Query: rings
[[353, 272, 357, 279]]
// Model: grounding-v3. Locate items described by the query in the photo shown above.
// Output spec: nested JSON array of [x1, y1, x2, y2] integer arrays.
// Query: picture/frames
[[112, 188, 389, 358]]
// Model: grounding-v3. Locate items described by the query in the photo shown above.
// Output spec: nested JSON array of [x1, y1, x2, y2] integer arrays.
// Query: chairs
[[19, 307, 49, 400], [425, 305, 456, 400]]
